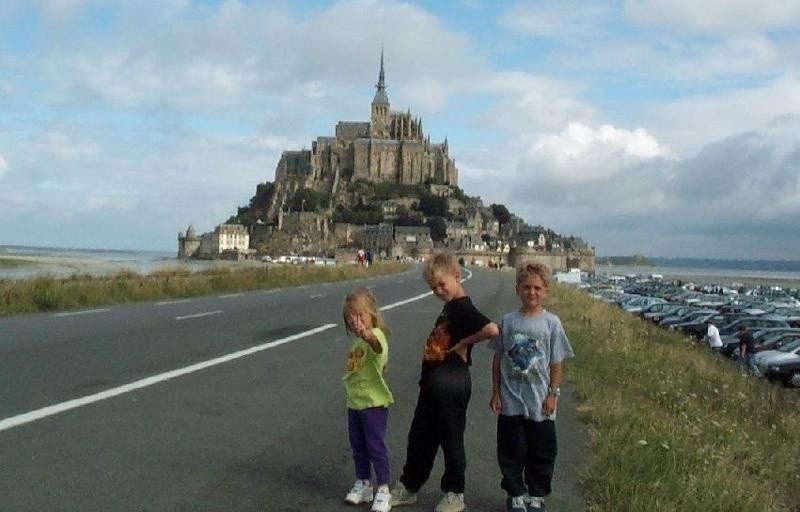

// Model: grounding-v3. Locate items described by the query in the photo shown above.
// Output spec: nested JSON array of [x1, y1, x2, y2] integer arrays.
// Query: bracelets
[[548, 386, 560, 396]]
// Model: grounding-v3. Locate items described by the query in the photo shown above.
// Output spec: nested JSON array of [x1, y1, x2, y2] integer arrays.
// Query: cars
[[554, 266, 800, 391]]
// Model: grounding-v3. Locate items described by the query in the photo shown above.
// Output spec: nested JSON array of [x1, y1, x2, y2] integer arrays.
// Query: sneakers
[[434, 491, 466, 512], [390, 479, 417, 507], [505, 493, 547, 512], [370, 486, 392, 512], [344, 478, 373, 505]]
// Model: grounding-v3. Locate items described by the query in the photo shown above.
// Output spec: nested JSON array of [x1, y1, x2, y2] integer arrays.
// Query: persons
[[357, 245, 364, 266], [366, 247, 373, 266], [498, 255, 504, 271], [390, 250, 502, 511], [488, 259, 576, 512], [703, 318, 723, 356], [341, 286, 396, 512], [488, 255, 494, 273], [732, 323, 763, 380]]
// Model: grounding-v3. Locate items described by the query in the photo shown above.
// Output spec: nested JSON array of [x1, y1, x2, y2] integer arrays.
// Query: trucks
[[261, 254, 271, 263]]
